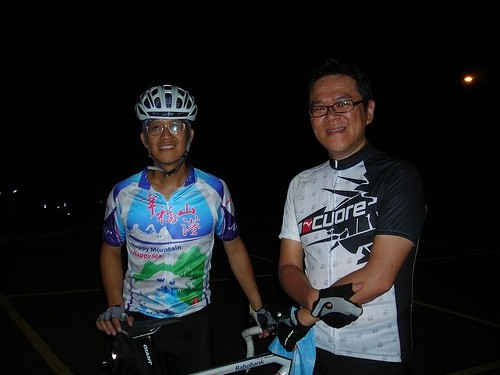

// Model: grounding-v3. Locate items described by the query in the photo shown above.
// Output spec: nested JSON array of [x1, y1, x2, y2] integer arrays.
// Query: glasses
[[308, 100, 363, 117], [145, 121, 187, 136]]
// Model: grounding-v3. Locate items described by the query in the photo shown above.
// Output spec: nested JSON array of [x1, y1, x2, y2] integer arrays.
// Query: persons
[[277, 57, 429, 375], [94, 83, 278, 375]]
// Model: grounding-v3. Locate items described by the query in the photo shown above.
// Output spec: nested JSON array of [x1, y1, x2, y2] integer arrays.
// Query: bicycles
[[99, 313, 312, 375]]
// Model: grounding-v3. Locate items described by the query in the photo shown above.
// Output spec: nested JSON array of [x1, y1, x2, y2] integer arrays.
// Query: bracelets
[[106, 304, 122, 311]]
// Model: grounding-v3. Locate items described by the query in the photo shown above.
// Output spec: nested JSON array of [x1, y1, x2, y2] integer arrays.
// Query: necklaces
[[152, 156, 185, 176]]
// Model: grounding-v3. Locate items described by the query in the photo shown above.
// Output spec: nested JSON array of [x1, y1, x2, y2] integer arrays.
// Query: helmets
[[135, 85, 197, 121]]
